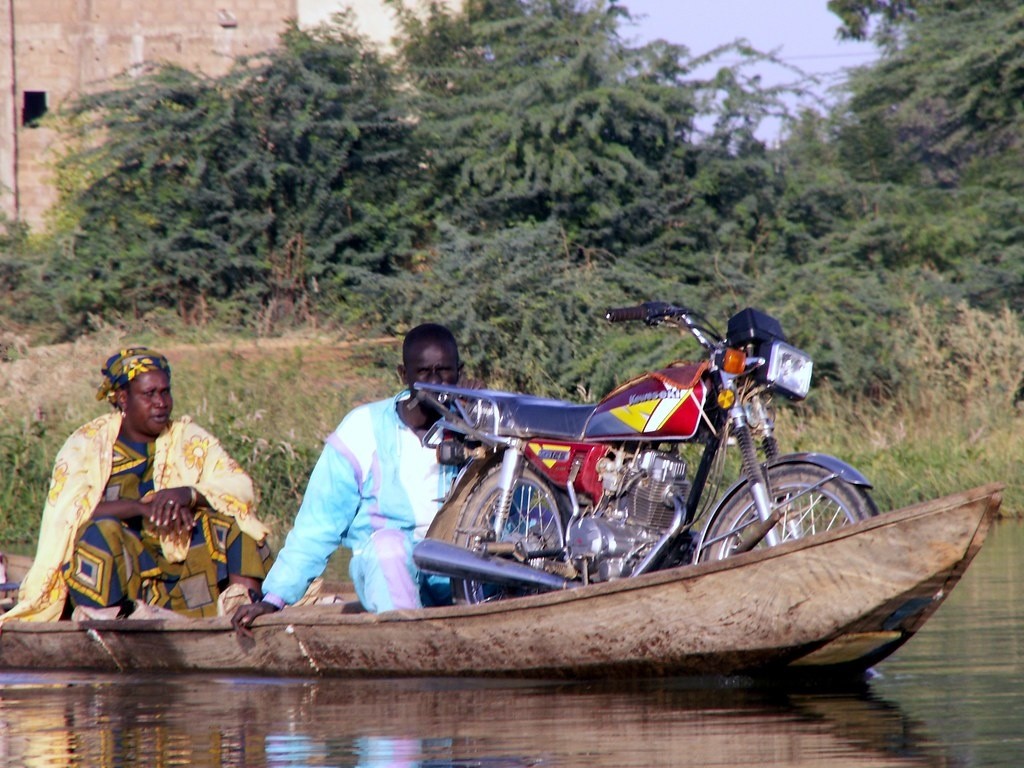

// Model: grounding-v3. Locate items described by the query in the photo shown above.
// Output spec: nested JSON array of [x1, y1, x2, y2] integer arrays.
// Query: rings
[[166, 499, 175, 506]]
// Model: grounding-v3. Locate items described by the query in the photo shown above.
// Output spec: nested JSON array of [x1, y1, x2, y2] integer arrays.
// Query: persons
[[33, 346, 276, 621], [230, 321, 489, 639]]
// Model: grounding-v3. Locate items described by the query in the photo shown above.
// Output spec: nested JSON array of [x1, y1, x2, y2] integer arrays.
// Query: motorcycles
[[404, 298, 880, 607]]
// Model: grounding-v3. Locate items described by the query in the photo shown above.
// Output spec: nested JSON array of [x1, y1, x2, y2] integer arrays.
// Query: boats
[[0, 482, 1005, 676]]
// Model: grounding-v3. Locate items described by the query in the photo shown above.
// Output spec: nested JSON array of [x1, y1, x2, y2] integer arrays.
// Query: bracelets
[[187, 486, 197, 508]]
